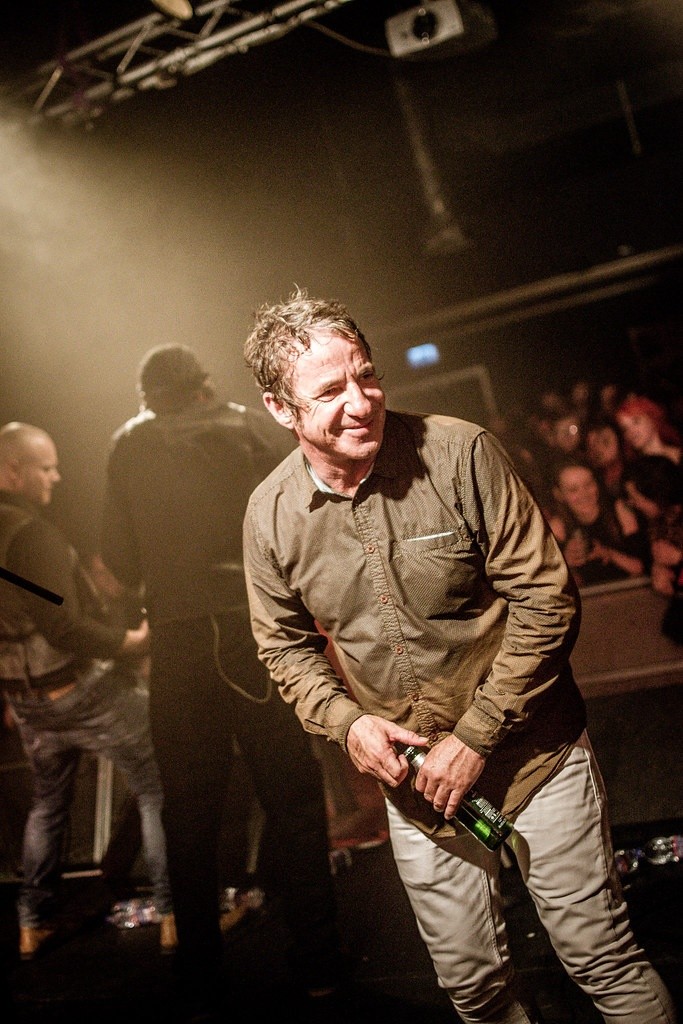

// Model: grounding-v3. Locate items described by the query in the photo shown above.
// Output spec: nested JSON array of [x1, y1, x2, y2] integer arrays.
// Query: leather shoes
[[158, 907, 247, 954], [18, 922, 52, 961]]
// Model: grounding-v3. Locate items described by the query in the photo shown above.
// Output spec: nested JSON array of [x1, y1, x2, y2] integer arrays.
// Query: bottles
[[403, 746, 515, 852]]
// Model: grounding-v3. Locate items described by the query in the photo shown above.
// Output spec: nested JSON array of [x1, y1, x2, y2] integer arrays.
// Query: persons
[[243, 280, 677, 1022], [1, 420, 178, 953], [96, 343, 345, 996], [493, 376, 682, 641]]
